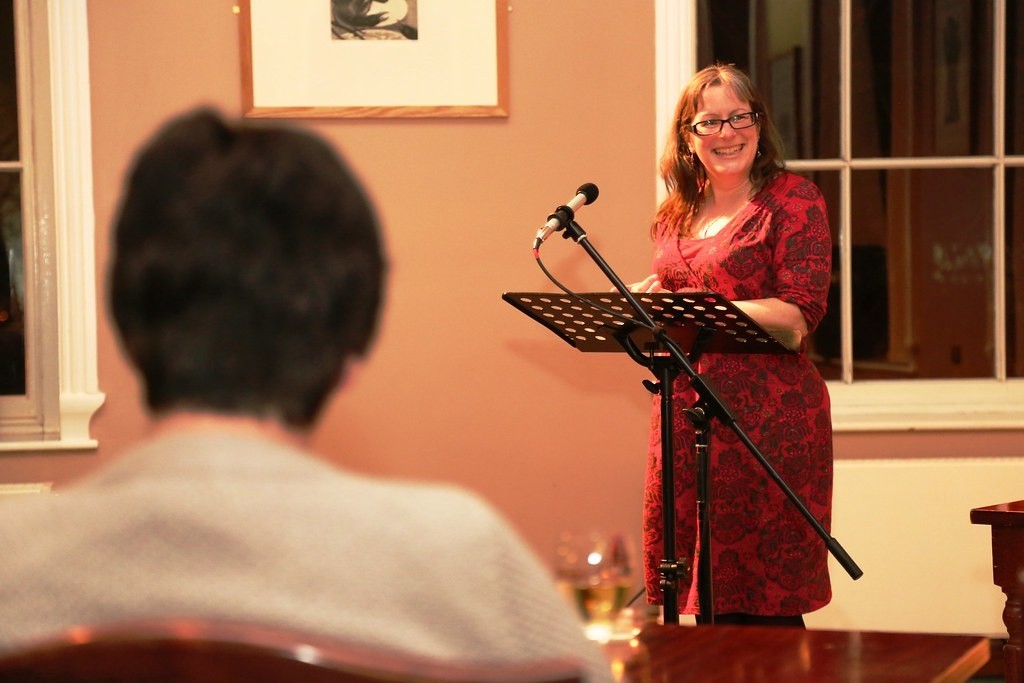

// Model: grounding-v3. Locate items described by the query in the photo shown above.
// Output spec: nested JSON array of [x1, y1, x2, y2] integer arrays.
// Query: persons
[[609, 65, 833, 630], [0, 95, 626, 682]]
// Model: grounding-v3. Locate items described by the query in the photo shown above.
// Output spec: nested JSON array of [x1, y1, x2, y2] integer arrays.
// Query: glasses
[[685, 112, 758, 136]]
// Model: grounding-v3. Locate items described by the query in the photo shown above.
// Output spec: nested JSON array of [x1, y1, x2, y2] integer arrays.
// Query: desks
[[572, 624, 992, 683]]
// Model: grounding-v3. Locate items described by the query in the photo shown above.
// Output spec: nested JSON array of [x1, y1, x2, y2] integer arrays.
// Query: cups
[[573, 526, 633, 630]]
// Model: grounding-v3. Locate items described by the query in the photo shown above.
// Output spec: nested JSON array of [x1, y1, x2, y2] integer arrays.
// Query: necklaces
[[702, 184, 747, 238]]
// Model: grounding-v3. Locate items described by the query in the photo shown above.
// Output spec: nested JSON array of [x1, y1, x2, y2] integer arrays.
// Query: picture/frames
[[931, 0, 981, 156], [758, 46, 802, 158], [236, 0, 511, 121]]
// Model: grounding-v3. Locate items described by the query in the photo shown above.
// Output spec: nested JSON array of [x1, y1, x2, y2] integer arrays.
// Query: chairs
[[1, 613, 590, 683]]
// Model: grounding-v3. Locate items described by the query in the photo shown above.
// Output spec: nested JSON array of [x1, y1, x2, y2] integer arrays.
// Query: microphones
[[533, 183, 600, 251]]
